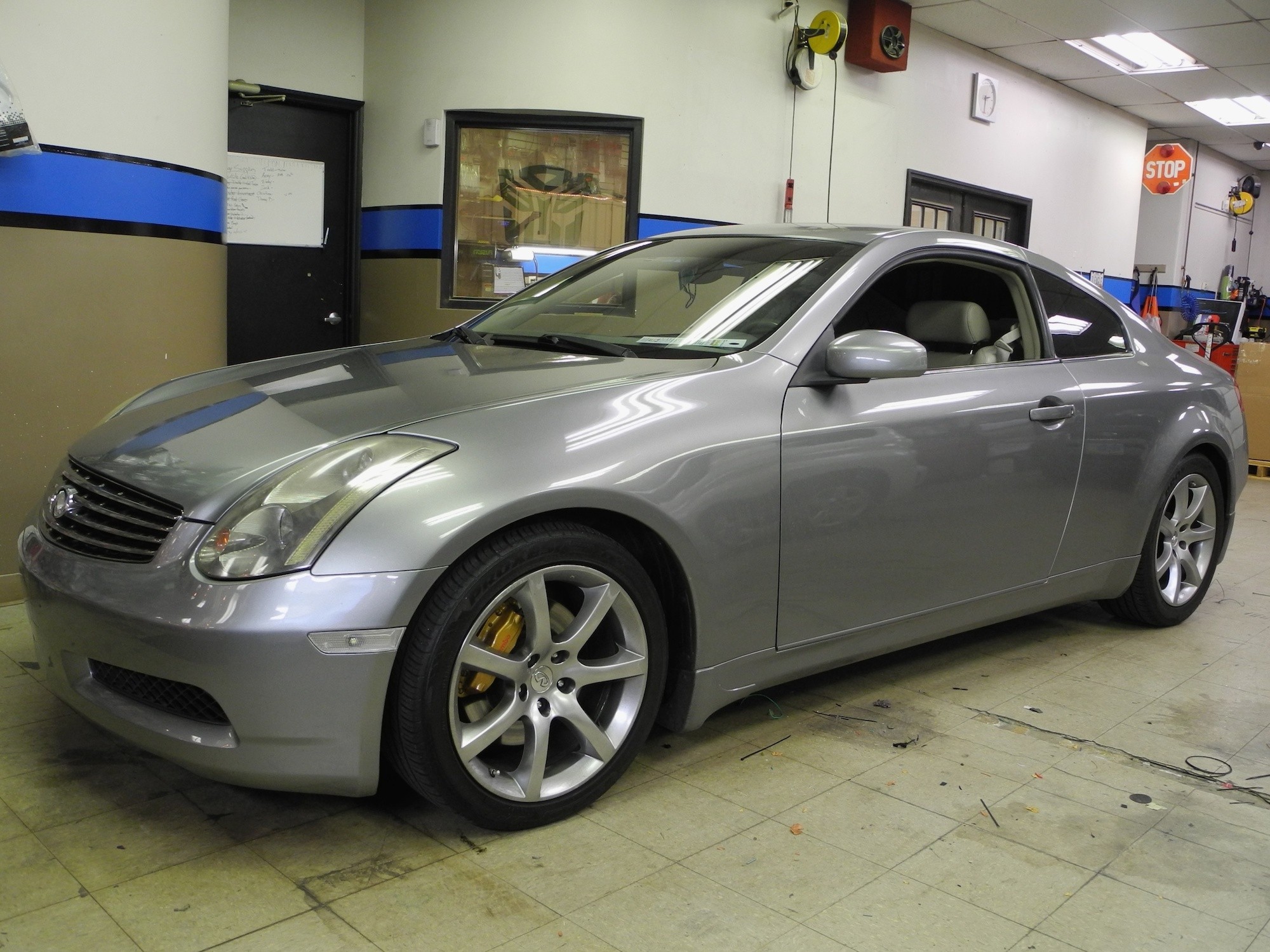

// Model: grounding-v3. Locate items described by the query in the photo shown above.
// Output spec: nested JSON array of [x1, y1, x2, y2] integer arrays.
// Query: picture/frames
[[440, 110, 642, 318]]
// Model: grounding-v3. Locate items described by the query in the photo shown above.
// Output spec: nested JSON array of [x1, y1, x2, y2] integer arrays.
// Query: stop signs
[[1144, 143, 1194, 195]]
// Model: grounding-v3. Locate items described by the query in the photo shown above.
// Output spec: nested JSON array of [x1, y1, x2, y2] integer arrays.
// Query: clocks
[[971, 72, 1000, 123]]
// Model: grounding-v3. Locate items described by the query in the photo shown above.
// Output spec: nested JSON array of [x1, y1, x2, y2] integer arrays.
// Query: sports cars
[[18, 223, 1252, 826]]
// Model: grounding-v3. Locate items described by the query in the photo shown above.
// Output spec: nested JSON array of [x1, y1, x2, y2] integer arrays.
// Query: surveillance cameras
[[1253, 139, 1265, 152]]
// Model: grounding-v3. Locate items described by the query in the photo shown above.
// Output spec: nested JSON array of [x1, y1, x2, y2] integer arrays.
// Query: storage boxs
[[1234, 340, 1270, 462]]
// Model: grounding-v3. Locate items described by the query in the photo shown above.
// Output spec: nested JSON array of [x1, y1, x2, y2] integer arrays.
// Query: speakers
[[846, 1, 913, 74]]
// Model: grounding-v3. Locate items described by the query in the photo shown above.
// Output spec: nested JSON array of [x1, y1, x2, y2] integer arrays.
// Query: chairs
[[905, 297, 993, 370]]
